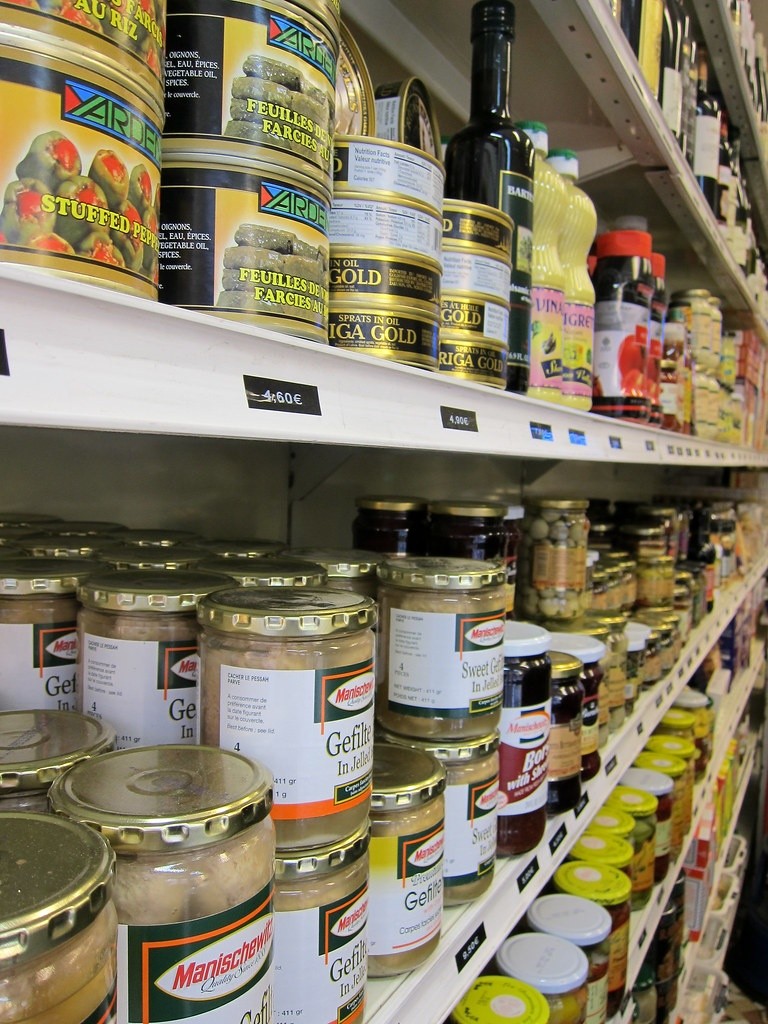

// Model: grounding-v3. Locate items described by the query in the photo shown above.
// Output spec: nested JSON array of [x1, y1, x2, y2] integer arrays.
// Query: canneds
[[0, 0, 768, 1023]]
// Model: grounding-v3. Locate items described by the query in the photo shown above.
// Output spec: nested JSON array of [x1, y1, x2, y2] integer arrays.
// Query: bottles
[[444, 0, 730, 433]]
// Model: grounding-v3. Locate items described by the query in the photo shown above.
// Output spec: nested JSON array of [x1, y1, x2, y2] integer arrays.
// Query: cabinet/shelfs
[[1, 1, 768, 1024]]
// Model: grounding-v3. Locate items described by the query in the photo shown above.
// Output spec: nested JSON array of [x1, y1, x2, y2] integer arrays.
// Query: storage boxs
[[676, 835, 749, 1024]]
